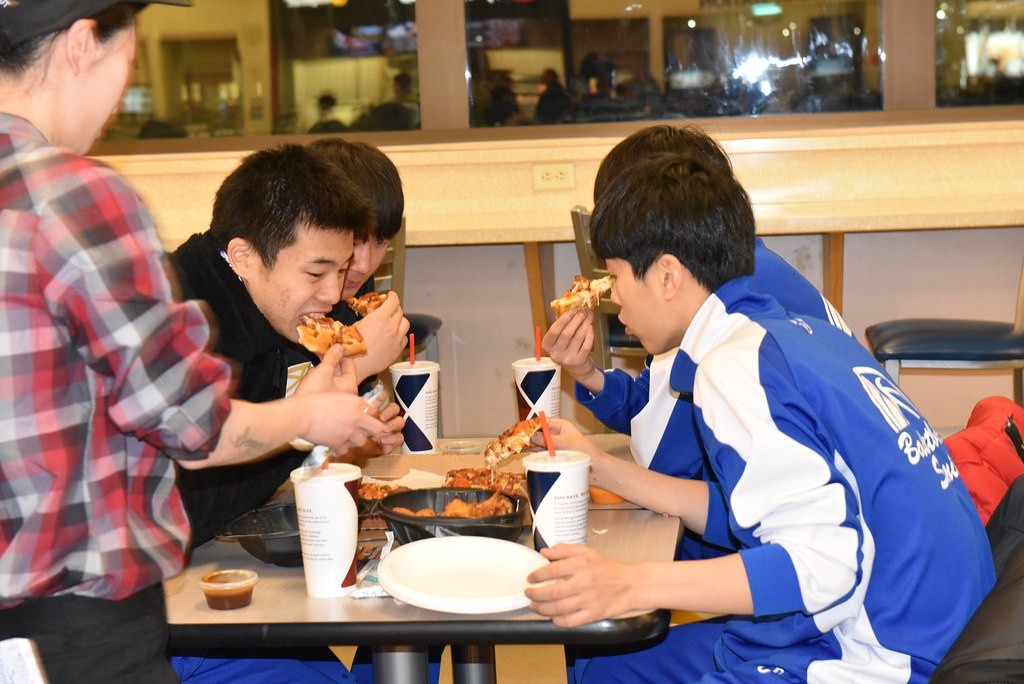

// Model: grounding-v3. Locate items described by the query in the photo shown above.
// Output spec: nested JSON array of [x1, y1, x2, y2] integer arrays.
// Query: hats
[[0, 0, 194, 51]]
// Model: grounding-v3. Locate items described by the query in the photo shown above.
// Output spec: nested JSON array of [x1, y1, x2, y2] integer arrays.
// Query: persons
[[486, 76, 518, 125], [138, 121, 186, 138], [523, 152, 996, 684], [541, 125, 856, 561], [165, 142, 441, 684], [536, 69, 570, 124], [309, 73, 420, 132], [0, 0, 384, 684]]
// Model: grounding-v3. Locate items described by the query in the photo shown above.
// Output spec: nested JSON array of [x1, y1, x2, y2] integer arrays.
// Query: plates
[[377, 535, 557, 615]]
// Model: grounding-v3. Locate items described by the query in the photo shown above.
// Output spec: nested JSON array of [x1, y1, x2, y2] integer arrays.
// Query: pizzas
[[484, 416, 551, 468], [298, 314, 367, 356], [357, 469, 529, 499], [347, 292, 389, 317], [550, 274, 613, 317]]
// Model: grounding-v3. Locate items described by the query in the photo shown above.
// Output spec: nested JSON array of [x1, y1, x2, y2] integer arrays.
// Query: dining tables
[[163, 433, 683, 684]]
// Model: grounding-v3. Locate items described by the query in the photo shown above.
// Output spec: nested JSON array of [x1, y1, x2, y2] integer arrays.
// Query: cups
[[522, 449, 593, 554], [388, 359, 440, 455], [198, 570, 260, 610], [511, 357, 562, 431], [290, 463, 363, 599]]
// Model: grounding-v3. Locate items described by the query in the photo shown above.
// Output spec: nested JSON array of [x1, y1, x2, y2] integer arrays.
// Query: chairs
[[372, 217, 443, 440], [571, 205, 650, 434], [865, 248, 1024, 409]]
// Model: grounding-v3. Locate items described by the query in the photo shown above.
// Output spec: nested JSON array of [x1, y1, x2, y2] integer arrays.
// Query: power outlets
[[532, 161, 575, 192]]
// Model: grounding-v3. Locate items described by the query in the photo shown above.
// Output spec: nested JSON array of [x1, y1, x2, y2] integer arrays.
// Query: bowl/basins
[[377, 485, 527, 547], [214, 499, 304, 568]]
